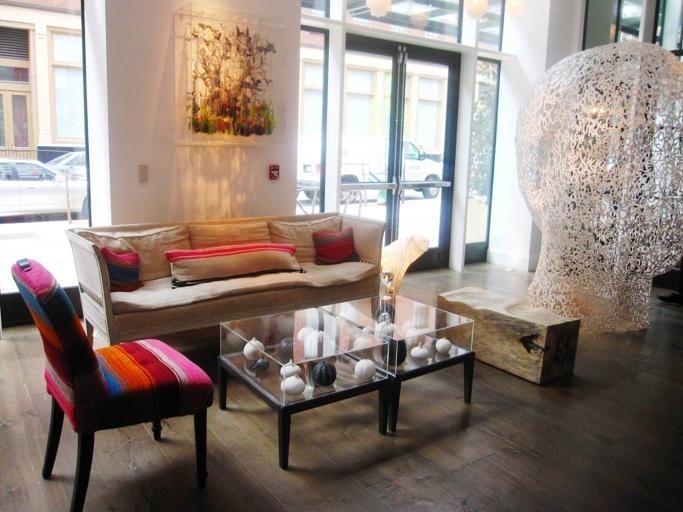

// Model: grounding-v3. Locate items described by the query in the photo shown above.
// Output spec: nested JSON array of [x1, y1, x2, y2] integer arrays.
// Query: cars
[[0, 150, 89, 217]]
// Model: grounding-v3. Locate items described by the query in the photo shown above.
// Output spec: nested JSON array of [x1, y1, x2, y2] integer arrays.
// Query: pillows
[[73, 216, 360, 291]]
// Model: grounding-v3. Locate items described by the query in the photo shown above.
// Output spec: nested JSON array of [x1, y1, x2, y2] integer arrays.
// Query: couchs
[[65, 211, 388, 351]]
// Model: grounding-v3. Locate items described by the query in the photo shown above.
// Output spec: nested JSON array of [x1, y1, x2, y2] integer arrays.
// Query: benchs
[[436, 285, 582, 386]]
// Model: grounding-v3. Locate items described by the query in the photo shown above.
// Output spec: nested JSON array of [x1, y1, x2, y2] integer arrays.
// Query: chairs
[[10, 257, 216, 511]]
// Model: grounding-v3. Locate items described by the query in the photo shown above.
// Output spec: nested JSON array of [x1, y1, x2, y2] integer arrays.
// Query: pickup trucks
[[309, 131, 443, 201]]
